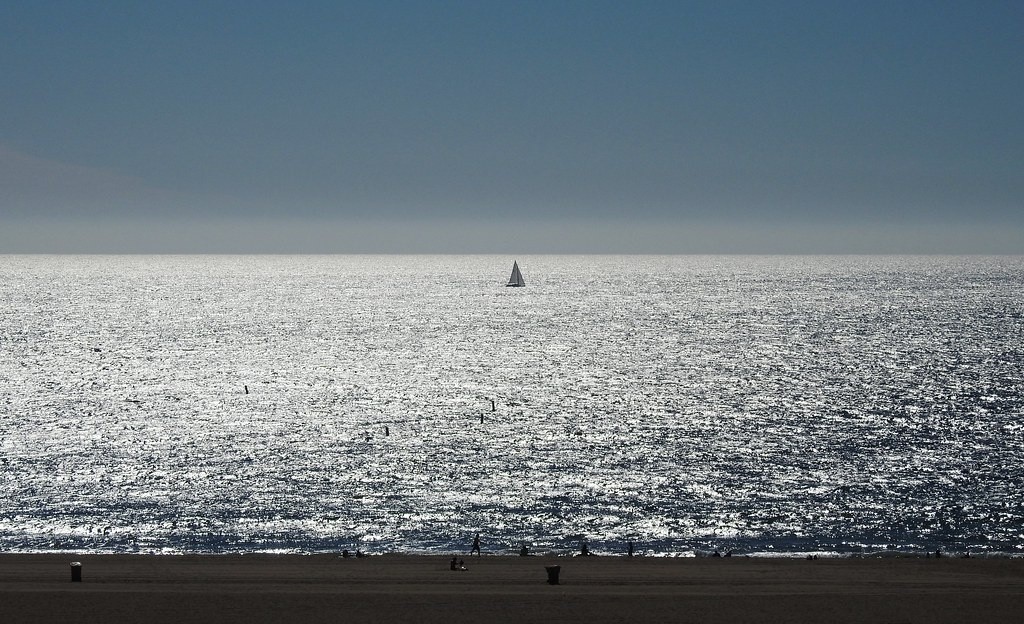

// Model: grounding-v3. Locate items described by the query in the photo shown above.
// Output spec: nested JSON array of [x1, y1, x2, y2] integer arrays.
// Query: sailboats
[[505, 260, 526, 288]]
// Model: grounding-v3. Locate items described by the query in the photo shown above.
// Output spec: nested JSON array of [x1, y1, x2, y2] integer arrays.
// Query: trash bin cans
[[69, 561, 82, 581], [544, 565, 561, 585]]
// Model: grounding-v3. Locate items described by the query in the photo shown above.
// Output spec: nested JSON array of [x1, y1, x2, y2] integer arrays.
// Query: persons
[[713, 551, 720, 557], [582, 545, 588, 555], [520, 546, 528, 556], [451, 558, 460, 570], [470, 534, 481, 557], [629, 542, 633, 556]]
